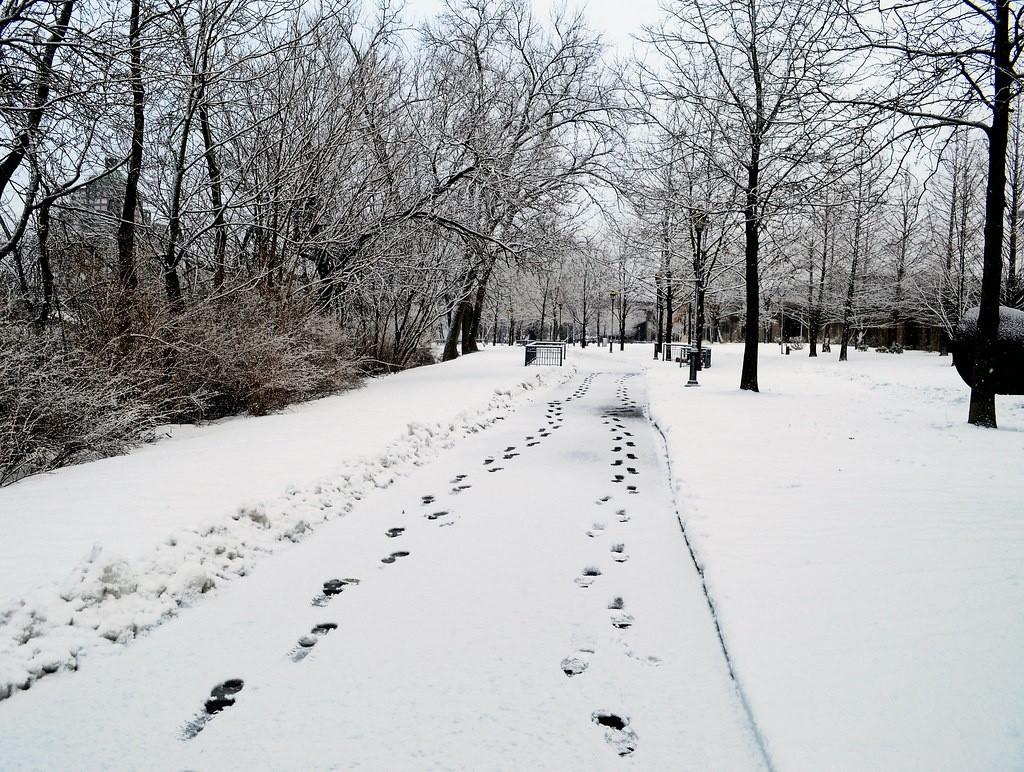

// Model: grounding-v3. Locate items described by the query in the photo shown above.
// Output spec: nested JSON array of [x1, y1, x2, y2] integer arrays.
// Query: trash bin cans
[[602, 338, 607, 347]]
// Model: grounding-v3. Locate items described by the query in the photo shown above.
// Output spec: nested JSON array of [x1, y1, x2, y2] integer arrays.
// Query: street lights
[[653, 272, 661, 359], [688, 201, 708, 385], [609, 291, 616, 353], [558, 301, 563, 341]]
